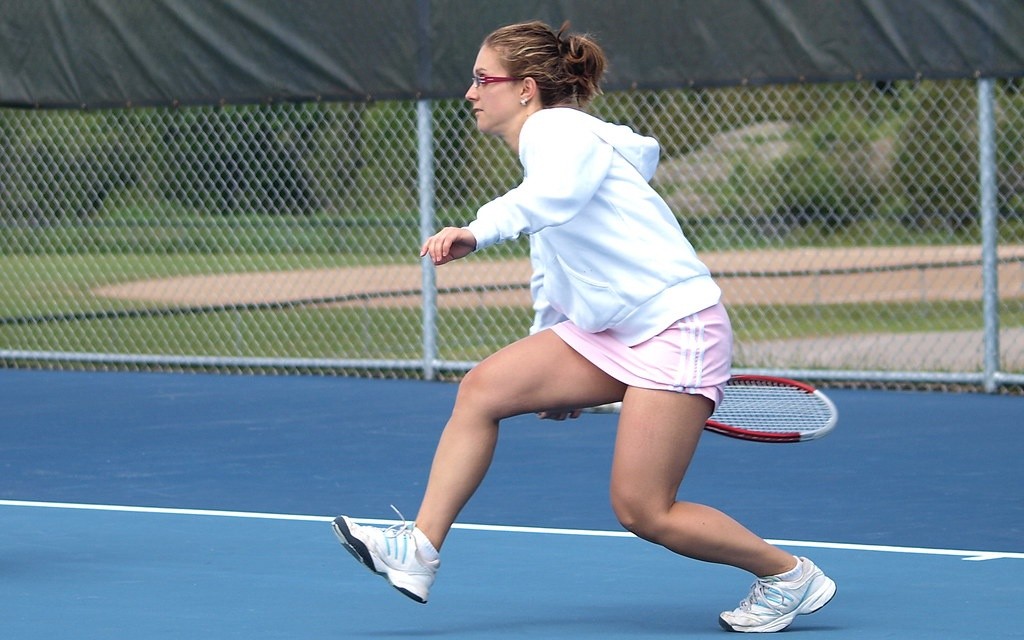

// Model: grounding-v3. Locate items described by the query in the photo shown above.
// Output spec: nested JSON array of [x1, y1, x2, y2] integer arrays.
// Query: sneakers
[[719, 556, 837, 633], [331, 504, 441, 605]]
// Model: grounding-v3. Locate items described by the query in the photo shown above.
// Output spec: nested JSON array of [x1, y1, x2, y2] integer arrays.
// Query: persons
[[332, 19, 839, 633]]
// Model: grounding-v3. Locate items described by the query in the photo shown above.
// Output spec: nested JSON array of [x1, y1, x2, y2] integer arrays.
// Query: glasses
[[472, 75, 525, 86]]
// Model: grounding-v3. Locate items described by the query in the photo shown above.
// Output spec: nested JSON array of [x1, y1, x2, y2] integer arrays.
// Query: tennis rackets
[[578, 373, 838, 442]]
